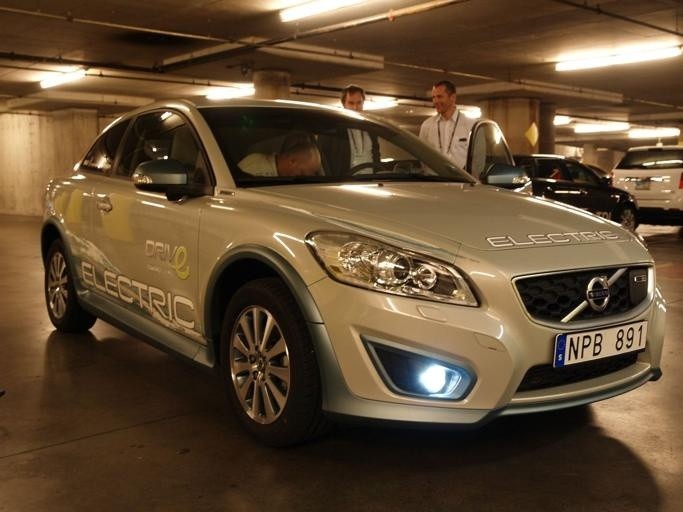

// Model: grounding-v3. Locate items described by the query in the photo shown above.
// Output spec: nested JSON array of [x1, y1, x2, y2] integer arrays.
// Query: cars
[[38, 95, 667, 452], [346, 154, 639, 231]]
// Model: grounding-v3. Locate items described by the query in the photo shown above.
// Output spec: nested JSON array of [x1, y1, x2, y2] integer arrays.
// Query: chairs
[[168, 126, 325, 183]]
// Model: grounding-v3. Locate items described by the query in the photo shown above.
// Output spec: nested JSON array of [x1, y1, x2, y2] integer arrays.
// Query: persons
[[225, 132, 321, 178], [336, 83, 380, 175], [419, 79, 479, 179]]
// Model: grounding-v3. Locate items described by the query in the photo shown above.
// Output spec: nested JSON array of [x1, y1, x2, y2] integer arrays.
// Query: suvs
[[608, 145, 683, 227]]
